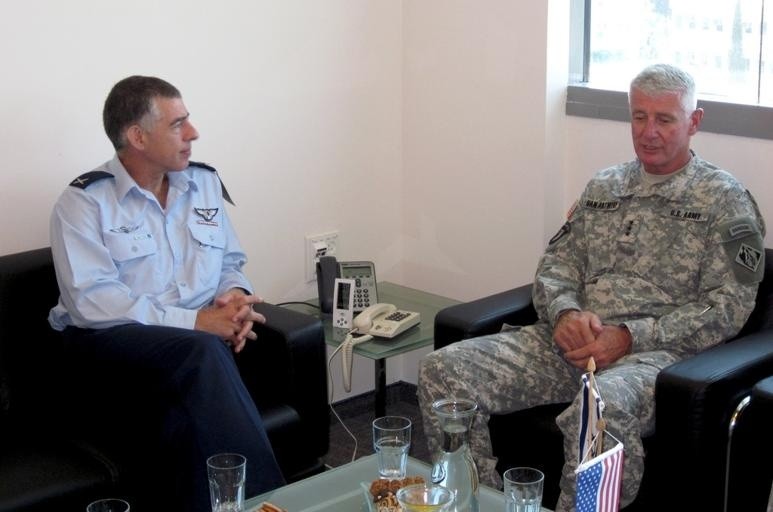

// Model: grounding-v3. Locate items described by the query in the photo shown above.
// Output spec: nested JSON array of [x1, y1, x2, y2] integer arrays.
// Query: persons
[[412, 62, 766, 512], [43, 74, 289, 512]]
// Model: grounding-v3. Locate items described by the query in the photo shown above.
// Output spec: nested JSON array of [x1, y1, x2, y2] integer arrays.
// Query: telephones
[[316, 256, 421, 338]]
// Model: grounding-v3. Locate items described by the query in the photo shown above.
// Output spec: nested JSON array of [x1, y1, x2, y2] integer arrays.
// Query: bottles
[[397, 484, 454, 511], [430, 399, 479, 512]]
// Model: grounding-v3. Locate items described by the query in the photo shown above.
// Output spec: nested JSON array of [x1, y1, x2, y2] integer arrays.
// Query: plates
[[361, 472, 425, 512]]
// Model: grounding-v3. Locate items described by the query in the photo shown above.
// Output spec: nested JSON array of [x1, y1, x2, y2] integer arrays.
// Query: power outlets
[[303, 231, 338, 283]]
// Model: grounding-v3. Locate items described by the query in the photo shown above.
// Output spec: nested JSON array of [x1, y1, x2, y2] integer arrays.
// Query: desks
[[274, 281, 466, 448], [216, 446, 555, 511]]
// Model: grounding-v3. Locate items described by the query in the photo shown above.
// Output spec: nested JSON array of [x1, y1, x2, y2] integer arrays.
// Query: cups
[[503, 467, 544, 512], [87, 499, 130, 512], [207, 454, 246, 511], [371, 415, 411, 480]]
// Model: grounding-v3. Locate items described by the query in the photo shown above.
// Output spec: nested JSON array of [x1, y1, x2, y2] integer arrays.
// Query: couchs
[[0, 246, 332, 511], [433, 247, 772, 512]]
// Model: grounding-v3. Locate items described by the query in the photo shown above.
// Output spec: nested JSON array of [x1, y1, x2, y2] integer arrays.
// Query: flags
[[573, 374, 606, 461], [573, 441, 625, 512]]
